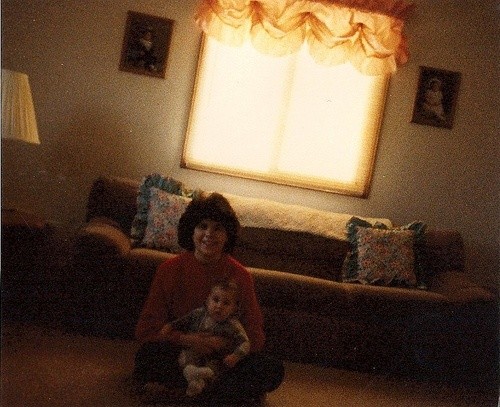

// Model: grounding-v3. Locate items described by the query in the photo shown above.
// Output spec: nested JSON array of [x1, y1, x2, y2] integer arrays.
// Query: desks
[[0, 209, 72, 320]]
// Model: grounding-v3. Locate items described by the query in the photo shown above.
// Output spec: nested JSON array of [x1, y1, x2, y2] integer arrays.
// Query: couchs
[[72, 175, 500, 380]]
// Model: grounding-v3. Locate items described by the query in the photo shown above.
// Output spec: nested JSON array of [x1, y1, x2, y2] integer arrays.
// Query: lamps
[[1, 69, 41, 144]]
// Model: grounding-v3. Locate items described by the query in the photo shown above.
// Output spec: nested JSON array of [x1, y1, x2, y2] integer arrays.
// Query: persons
[[161, 278, 251, 396], [134, 193, 285, 407]]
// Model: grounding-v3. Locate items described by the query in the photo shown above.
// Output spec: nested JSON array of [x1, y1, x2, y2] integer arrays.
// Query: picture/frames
[[410, 66, 462, 130], [119, 11, 175, 79]]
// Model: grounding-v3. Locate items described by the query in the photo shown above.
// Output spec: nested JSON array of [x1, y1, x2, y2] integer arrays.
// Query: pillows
[[130, 173, 206, 254], [342, 218, 428, 289]]
